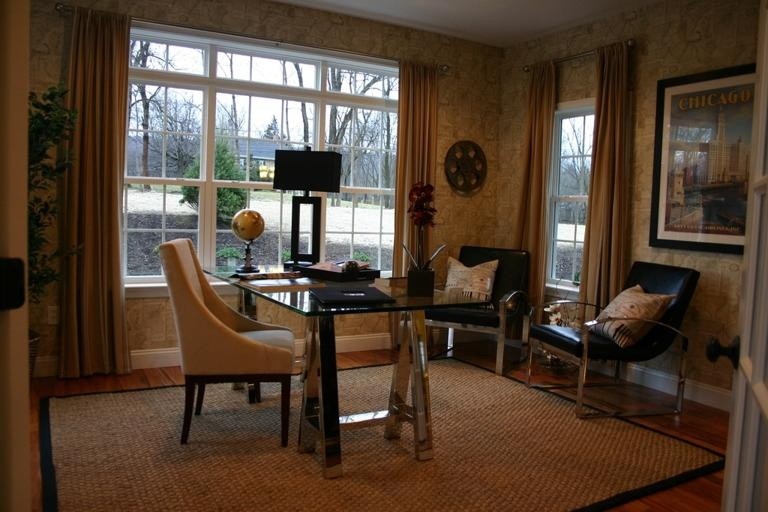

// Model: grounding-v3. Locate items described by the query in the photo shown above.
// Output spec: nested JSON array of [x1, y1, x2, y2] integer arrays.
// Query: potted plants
[[398, 180, 448, 298], [27, 81, 81, 395]]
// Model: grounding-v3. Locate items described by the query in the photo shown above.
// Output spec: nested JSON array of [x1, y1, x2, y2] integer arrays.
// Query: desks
[[199, 250, 494, 481]]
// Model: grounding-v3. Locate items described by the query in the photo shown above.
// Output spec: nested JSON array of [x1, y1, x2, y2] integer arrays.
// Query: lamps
[[272, 143, 343, 274]]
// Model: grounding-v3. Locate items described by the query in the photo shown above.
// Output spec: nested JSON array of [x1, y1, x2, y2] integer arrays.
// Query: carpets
[[39, 352, 727, 512]]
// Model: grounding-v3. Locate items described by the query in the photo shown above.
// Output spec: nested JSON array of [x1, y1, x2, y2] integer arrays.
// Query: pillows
[[588, 282, 677, 349], [441, 253, 498, 305]]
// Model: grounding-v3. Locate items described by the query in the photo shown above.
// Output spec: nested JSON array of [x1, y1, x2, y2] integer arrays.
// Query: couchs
[[158, 236, 300, 448], [423, 239, 532, 377], [525, 259, 702, 421]]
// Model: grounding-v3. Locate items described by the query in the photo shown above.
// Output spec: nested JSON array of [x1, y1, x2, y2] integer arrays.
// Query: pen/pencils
[[422, 242, 448, 271], [400, 242, 418, 267]]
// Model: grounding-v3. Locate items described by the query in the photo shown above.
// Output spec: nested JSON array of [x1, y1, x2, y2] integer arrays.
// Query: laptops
[[308, 287, 396, 305]]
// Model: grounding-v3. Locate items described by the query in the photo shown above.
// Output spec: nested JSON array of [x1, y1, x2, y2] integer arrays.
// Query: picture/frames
[[646, 62, 760, 255]]
